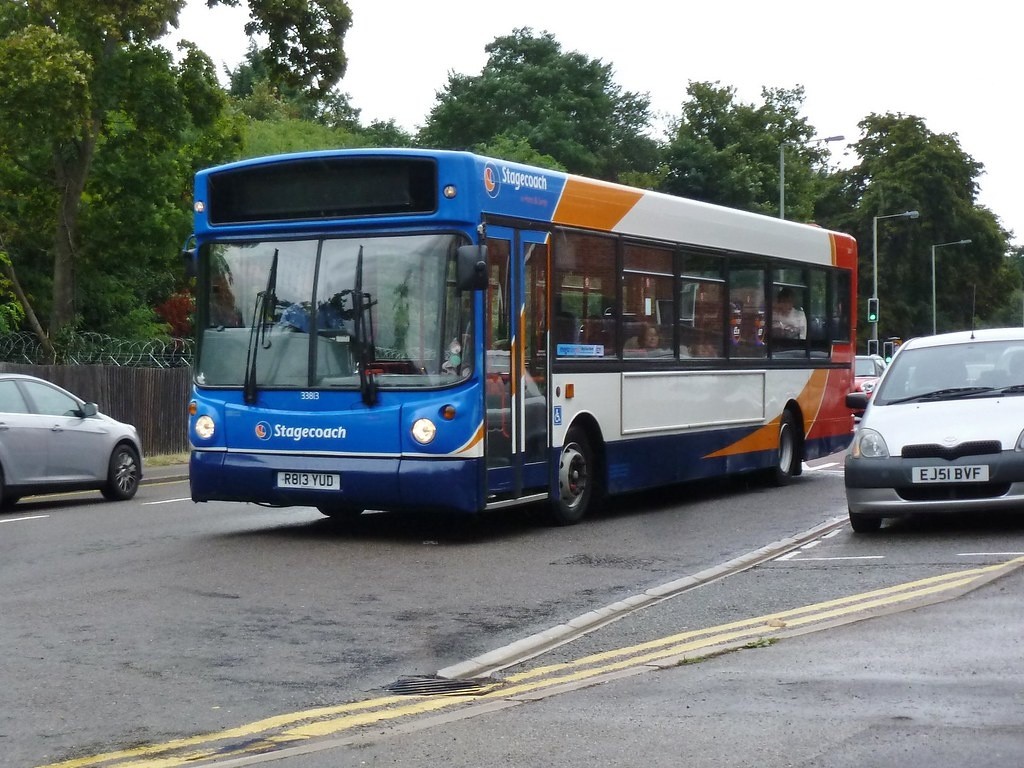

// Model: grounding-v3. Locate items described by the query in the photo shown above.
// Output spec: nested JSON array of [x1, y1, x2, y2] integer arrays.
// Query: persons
[[279, 270, 347, 333], [625, 314, 766, 358], [771, 287, 807, 340]]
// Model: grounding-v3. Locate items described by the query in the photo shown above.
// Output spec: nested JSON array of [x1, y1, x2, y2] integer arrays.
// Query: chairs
[[624, 337, 690, 359], [1008, 351, 1024, 385], [557, 312, 604, 345], [716, 302, 848, 360]]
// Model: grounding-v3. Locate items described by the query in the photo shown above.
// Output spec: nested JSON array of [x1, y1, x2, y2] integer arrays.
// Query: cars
[[843, 327, 1024, 531], [0, 373, 145, 512], [853, 354, 887, 418]]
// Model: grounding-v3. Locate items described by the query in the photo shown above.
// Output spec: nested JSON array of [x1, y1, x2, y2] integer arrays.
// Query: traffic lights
[[882, 342, 894, 364], [868, 298, 879, 323], [868, 340, 878, 356]]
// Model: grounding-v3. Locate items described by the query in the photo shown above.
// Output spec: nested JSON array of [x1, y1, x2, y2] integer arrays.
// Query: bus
[[183, 149, 861, 526]]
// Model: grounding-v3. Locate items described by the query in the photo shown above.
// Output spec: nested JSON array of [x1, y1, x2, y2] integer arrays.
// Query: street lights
[[930, 240, 973, 333], [779, 135, 845, 290], [871, 210, 920, 347]]
